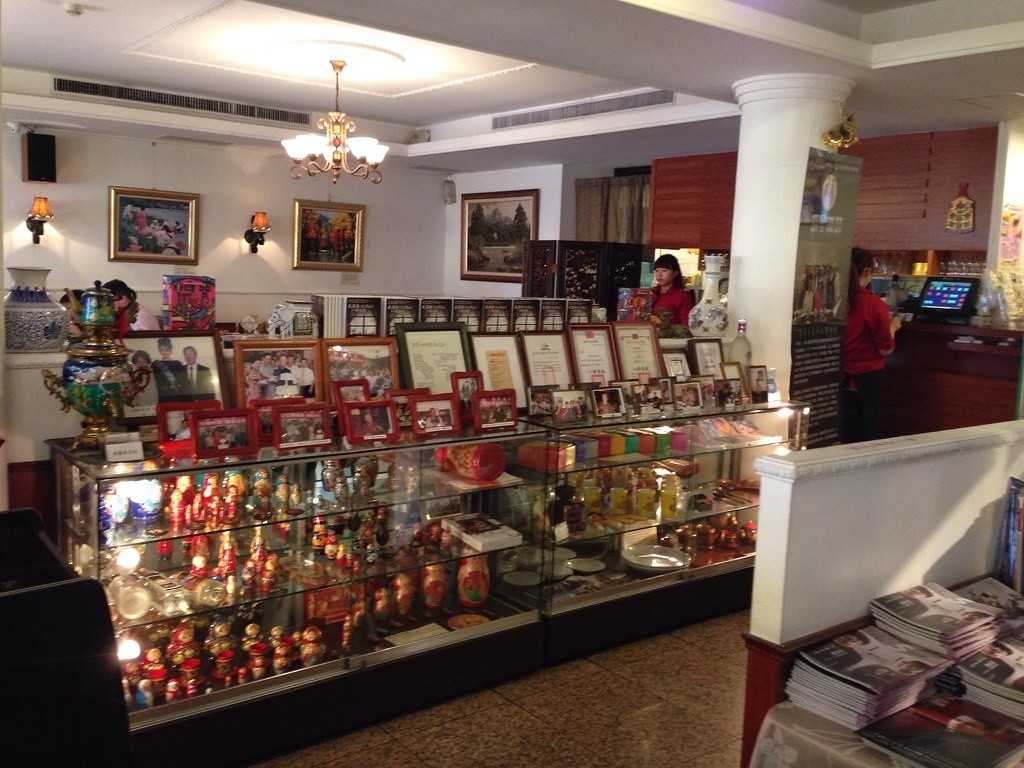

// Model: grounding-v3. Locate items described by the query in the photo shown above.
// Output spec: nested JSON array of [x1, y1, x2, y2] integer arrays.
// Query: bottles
[[886, 274, 901, 312], [99, 563, 227, 622], [977, 280, 1010, 320], [688, 256, 729, 337], [871, 250, 913, 276], [938, 260, 987, 277], [3, 266, 71, 353], [727, 319, 753, 404]]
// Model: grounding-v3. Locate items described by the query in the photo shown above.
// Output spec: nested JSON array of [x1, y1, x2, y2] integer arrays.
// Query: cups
[[629, 489, 656, 517], [595, 466, 681, 502], [604, 487, 628, 515], [888, 312, 913, 321], [583, 486, 602, 513], [659, 490, 680, 517]]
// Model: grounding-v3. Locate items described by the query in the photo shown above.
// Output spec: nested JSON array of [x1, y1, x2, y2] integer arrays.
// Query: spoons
[[712, 486, 753, 504]]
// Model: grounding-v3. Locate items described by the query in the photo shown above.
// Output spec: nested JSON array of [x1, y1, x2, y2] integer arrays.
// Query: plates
[[619, 544, 692, 574], [448, 613, 490, 630], [565, 558, 606, 572], [497, 546, 577, 586]]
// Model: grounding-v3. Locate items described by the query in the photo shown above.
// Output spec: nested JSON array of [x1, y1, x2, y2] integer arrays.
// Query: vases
[[2, 267, 70, 354], [688, 256, 729, 339]]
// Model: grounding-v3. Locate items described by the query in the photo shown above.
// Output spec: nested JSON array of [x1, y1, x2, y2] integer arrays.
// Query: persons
[[755, 379, 762, 402], [1000, 598, 1024, 620], [963, 642, 1016, 684], [682, 388, 696, 406], [462, 380, 470, 407], [103, 280, 160, 330], [555, 397, 587, 419], [121, 202, 146, 235], [531, 397, 553, 414], [140, 468, 328, 683], [703, 384, 713, 399], [842, 247, 901, 442], [873, 586, 932, 620], [798, 264, 835, 312], [130, 338, 446, 451], [653, 392, 659, 404], [886, 714, 1004, 768], [805, 629, 869, 671], [848, 660, 930, 689], [915, 610, 992, 631], [649, 254, 694, 337], [61, 290, 85, 335], [479, 397, 512, 423], [597, 393, 612, 413], [718, 384, 741, 405]]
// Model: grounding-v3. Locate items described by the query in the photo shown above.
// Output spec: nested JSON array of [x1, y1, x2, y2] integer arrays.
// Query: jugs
[[739, 520, 757, 545]]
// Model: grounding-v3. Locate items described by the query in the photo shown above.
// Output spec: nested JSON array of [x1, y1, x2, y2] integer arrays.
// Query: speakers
[[20, 133, 57, 184]]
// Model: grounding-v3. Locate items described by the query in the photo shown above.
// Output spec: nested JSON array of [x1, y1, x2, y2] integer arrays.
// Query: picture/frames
[[290, 198, 365, 271], [186, 407, 259, 461], [332, 377, 373, 435], [338, 403, 399, 444], [108, 329, 228, 423], [107, 185, 200, 268], [230, 338, 324, 408], [412, 391, 465, 431], [384, 386, 431, 430], [459, 188, 539, 282], [270, 402, 335, 452], [154, 399, 222, 450], [321, 335, 401, 406], [249, 394, 309, 448], [453, 370, 486, 422], [526, 341, 770, 425], [471, 388, 520, 432]]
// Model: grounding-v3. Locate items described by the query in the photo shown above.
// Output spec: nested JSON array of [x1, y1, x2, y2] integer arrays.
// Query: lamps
[[27, 197, 54, 244], [280, 59, 391, 185], [245, 211, 272, 253]]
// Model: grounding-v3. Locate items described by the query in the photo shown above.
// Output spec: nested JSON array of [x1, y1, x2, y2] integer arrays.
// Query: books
[[784, 477, 1024, 768]]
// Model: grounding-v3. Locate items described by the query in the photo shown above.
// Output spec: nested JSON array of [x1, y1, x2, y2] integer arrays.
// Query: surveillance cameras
[[10, 125, 19, 133]]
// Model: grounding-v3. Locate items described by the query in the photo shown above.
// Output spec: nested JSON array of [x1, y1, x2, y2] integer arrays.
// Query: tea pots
[[64, 280, 137, 347]]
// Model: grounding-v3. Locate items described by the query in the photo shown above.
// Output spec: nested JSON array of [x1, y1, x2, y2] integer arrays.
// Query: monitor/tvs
[[913, 277, 980, 325]]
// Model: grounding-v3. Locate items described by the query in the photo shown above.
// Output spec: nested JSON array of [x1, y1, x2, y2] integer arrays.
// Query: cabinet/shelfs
[[46, 399, 814, 731]]
